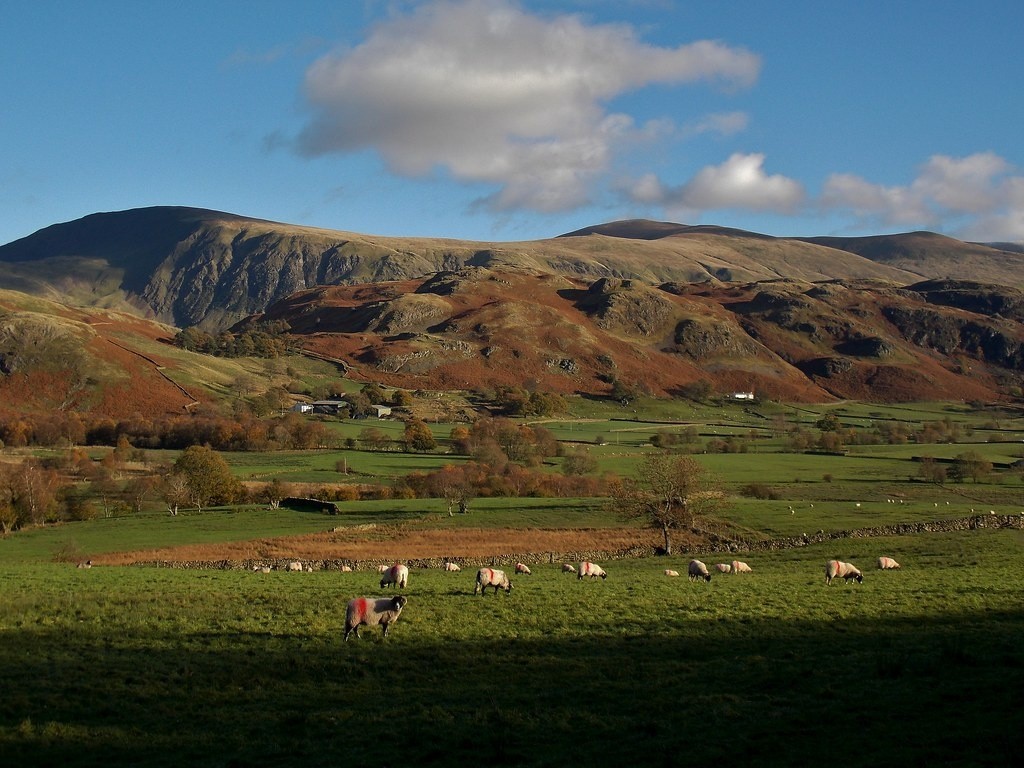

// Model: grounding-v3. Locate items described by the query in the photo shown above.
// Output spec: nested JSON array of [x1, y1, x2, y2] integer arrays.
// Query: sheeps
[[71, 495, 1021, 643]]
